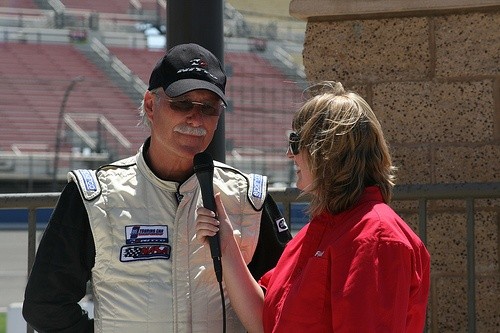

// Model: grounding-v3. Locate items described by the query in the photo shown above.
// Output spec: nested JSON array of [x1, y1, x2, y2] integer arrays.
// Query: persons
[[195, 82, 434, 332], [21, 43, 293, 333]]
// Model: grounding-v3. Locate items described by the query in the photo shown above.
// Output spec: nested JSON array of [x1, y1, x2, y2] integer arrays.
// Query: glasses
[[151, 94, 226, 117], [288, 132, 302, 155]]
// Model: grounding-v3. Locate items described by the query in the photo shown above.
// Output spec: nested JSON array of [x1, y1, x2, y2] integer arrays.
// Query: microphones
[[193, 152, 223, 281]]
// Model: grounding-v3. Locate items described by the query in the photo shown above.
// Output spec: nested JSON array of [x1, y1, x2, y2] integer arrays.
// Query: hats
[[147, 43, 228, 107]]
